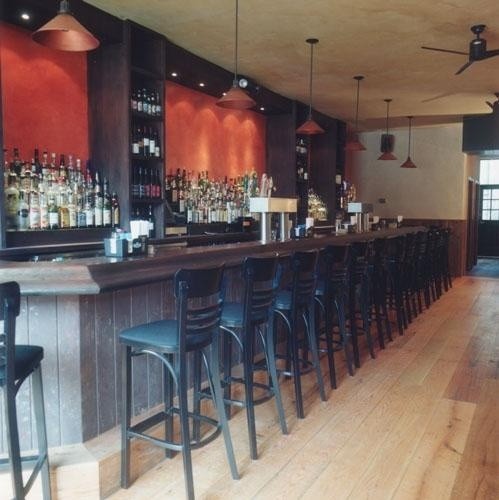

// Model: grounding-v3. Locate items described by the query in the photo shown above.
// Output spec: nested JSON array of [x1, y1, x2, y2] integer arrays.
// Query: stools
[[253, 247, 327, 419], [302, 242, 354, 391], [344, 237, 385, 361], [117, 259, 239, 499], [188, 251, 287, 459], [378, 225, 453, 342], [0, 281, 50, 500]]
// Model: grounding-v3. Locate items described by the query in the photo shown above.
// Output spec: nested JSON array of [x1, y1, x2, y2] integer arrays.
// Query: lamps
[[30, 0, 101, 55], [399, 116, 417, 169], [216, 1, 257, 112], [377, 98, 399, 161], [344, 75, 368, 152], [294, 38, 325, 135]]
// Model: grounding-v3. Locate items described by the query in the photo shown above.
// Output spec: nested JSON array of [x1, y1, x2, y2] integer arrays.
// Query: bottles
[[166, 168, 259, 223], [2, 147, 120, 230], [132, 86, 161, 237], [104, 231, 148, 257], [296, 138, 308, 180]]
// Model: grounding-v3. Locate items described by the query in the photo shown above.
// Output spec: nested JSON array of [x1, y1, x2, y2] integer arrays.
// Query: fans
[[484, 93, 499, 116], [420, 24, 499, 77]]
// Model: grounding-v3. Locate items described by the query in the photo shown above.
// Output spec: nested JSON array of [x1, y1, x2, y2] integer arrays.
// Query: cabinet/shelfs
[[266, 100, 313, 225], [85, 18, 167, 239]]
